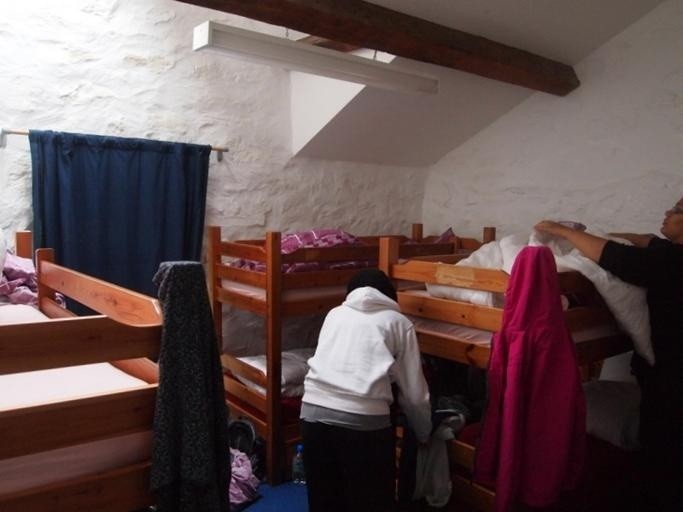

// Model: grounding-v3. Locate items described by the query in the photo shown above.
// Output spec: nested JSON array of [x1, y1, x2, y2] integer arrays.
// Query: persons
[[530, 194, 683, 512], [296, 267, 433, 511]]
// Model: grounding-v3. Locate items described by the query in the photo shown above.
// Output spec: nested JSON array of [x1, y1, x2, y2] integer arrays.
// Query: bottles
[[292, 445, 306, 486]]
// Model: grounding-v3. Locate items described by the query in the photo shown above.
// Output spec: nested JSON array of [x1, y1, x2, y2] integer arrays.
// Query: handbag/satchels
[[399, 390, 473, 450]]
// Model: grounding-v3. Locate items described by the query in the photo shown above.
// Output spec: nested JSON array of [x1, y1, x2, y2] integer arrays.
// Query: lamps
[[193, 20, 440, 95]]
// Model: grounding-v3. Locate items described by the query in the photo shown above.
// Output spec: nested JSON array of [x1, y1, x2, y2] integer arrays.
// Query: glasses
[[671, 205, 683, 215]]
